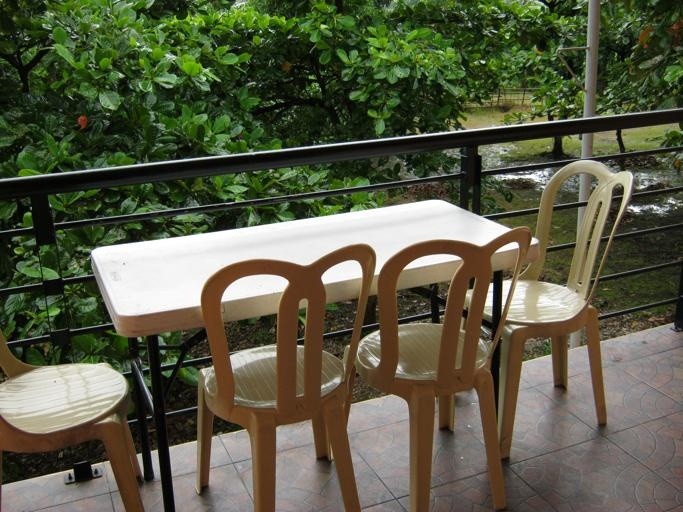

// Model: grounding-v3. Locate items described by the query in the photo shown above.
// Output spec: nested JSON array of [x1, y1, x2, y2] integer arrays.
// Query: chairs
[[460, 161, 633, 460], [342, 225, 532, 511], [1, 330, 143, 511], [194, 243, 376, 511]]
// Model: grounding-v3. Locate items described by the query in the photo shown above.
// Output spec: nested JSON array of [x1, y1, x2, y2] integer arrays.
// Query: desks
[[89, 199, 538, 511]]
